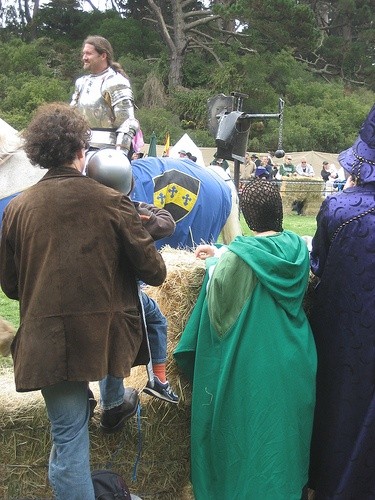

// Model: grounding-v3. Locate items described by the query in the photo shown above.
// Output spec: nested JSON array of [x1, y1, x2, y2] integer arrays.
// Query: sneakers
[[88, 397, 97, 419], [143, 374, 180, 405]]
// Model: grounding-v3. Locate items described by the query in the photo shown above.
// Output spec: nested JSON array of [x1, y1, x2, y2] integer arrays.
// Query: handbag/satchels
[[90, 470, 132, 500]]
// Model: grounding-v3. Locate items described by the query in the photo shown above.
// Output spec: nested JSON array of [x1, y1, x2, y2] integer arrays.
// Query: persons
[[132, 149, 348, 217], [310, 103, 375, 500], [85, 148, 180, 421], [0, 103, 166, 500], [69, 35, 140, 156], [172, 179, 318, 500]]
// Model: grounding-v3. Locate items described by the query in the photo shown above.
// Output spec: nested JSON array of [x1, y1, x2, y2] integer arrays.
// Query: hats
[[186, 152, 193, 156], [323, 161, 329, 165], [338, 103, 375, 183], [251, 153, 258, 159], [178, 150, 187, 156]]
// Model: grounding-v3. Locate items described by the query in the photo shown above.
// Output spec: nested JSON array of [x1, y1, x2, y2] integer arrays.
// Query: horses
[[0, 116, 241, 250]]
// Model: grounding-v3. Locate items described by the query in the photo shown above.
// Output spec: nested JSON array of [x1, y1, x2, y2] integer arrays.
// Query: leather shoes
[[99, 388, 139, 431]]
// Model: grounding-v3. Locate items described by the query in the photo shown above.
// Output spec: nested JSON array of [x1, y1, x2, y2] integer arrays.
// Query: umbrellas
[[147, 130, 157, 157], [162, 128, 170, 157]]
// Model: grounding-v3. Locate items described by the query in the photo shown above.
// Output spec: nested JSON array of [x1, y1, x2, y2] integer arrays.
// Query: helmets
[[86, 148, 135, 194]]
